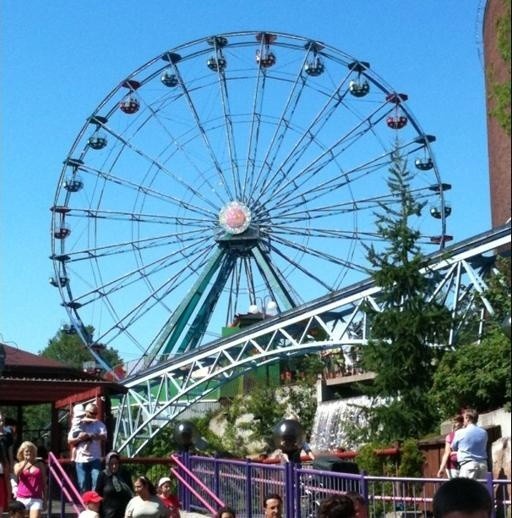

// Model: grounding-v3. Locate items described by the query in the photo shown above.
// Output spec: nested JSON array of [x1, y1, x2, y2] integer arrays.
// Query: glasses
[[86, 411, 98, 415]]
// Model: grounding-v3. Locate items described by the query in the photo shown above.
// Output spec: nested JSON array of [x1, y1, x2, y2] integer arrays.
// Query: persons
[[317, 493, 355, 518], [436, 414, 464, 478], [280, 363, 363, 384], [345, 491, 368, 518], [430, 476, 492, 518], [450, 408, 488, 487], [261, 493, 283, 518], [217, 506, 235, 518], [0, 402, 181, 518]]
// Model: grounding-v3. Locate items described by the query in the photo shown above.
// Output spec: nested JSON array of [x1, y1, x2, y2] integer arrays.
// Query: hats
[[158, 477, 172, 487], [72, 404, 86, 418], [82, 491, 105, 504]]
